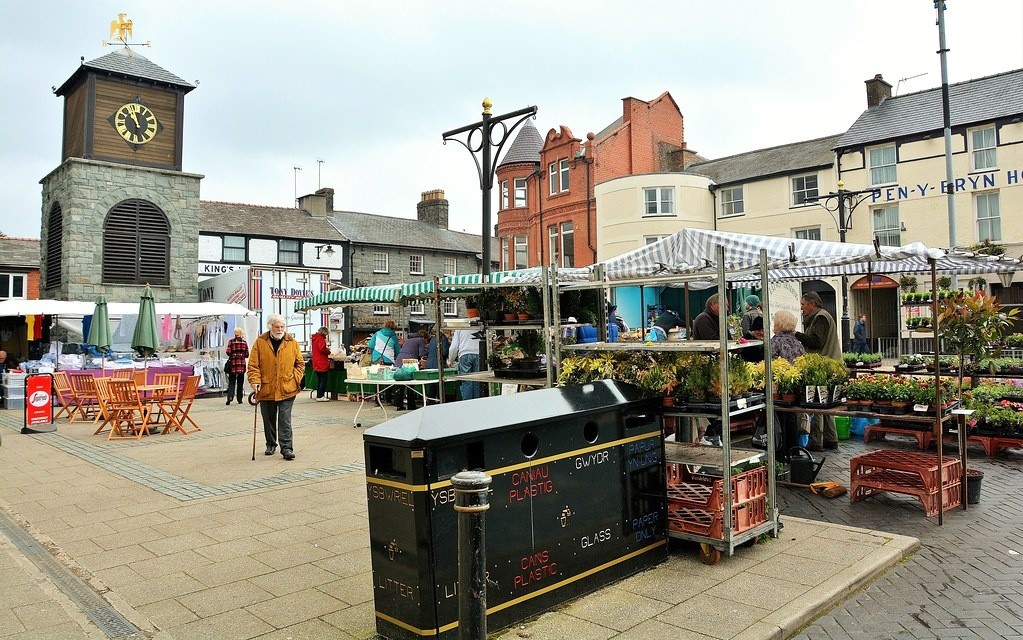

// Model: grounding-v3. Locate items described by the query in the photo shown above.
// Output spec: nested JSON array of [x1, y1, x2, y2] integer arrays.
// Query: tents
[[290, 265, 558, 409], [521, 227, 1023, 388], [0, 300, 259, 397]]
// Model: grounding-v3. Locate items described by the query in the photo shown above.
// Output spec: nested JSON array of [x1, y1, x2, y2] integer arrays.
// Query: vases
[[466, 309, 478, 318], [961, 468, 984, 504], [845, 397, 955, 415], [518, 313, 529, 319], [504, 314, 516, 320], [974, 396, 1023, 439]]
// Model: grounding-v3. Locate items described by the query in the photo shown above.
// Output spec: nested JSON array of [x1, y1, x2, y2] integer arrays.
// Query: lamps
[[997, 272, 1015, 288], [315, 241, 335, 259]]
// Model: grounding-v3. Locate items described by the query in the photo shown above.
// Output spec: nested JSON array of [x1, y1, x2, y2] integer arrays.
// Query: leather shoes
[[397, 406, 406, 410], [407, 405, 420, 410]]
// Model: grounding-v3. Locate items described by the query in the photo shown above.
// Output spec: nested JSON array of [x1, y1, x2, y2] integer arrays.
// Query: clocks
[[107, 95, 164, 152]]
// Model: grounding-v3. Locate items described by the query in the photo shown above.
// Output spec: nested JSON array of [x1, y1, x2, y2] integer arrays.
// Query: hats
[[745, 295, 759, 307]]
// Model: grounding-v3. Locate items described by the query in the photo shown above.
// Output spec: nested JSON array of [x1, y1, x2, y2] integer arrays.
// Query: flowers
[[465, 286, 540, 340], [962, 378, 1023, 429], [841, 374, 955, 404]]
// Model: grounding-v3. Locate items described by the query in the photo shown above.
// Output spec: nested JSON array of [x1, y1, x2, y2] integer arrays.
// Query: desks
[[850, 450, 961, 516], [77, 377, 129, 421], [116, 384, 174, 436], [305, 359, 377, 401], [344, 379, 439, 427], [57, 365, 206, 404]]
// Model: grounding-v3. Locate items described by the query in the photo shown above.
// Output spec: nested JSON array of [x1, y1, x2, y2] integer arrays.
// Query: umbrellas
[[131, 282, 161, 436], [86, 291, 114, 421]]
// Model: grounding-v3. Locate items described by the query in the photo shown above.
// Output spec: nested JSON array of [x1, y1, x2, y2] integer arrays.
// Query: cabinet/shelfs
[[551, 244, 779, 565], [433, 264, 598, 406]]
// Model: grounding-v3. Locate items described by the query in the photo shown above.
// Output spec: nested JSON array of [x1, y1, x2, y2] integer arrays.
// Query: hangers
[[191, 315, 221, 323]]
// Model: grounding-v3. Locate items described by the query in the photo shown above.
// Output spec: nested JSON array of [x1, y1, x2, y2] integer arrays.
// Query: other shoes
[[239, 401, 243, 404], [807, 447, 824, 451], [226, 398, 233, 405], [265, 447, 276, 455], [283, 452, 295, 459], [315, 396, 330, 401], [824, 442, 838, 449]]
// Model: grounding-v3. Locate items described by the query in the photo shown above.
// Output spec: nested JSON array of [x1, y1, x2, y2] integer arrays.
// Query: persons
[[740, 294, 764, 365], [792, 291, 840, 451], [368, 321, 401, 407], [648, 308, 692, 343], [771, 311, 807, 464], [394, 330, 428, 411], [425, 329, 452, 406], [246, 314, 305, 460], [609, 304, 619, 342], [852, 314, 871, 354], [0, 350, 7, 406], [446, 329, 485, 400], [311, 327, 331, 402], [225, 328, 249, 406], [693, 293, 734, 448]]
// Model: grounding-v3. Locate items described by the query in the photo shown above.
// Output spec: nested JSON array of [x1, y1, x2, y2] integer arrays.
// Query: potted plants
[[899, 276, 917, 293], [516, 329, 546, 369], [906, 317, 934, 327], [981, 357, 1023, 371], [902, 289, 973, 301], [899, 355, 923, 365], [924, 355, 959, 368], [935, 276, 951, 291], [1006, 332, 1023, 349], [968, 276, 986, 290], [842, 351, 882, 364], [552, 351, 849, 407]]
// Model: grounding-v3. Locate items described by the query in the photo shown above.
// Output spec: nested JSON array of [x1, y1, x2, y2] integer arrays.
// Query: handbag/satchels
[[798, 413, 810, 434], [370, 357, 384, 365], [224, 360, 232, 373], [751, 411, 783, 450]]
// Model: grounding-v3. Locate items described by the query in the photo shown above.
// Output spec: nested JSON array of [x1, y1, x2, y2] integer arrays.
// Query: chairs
[[50, 367, 201, 440]]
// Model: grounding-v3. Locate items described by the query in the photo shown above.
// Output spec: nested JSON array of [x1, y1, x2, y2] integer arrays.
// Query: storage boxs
[[413, 368, 456, 396], [347, 366, 395, 380], [668, 328, 686, 339], [29, 366, 54, 373], [1, 373, 27, 410]]
